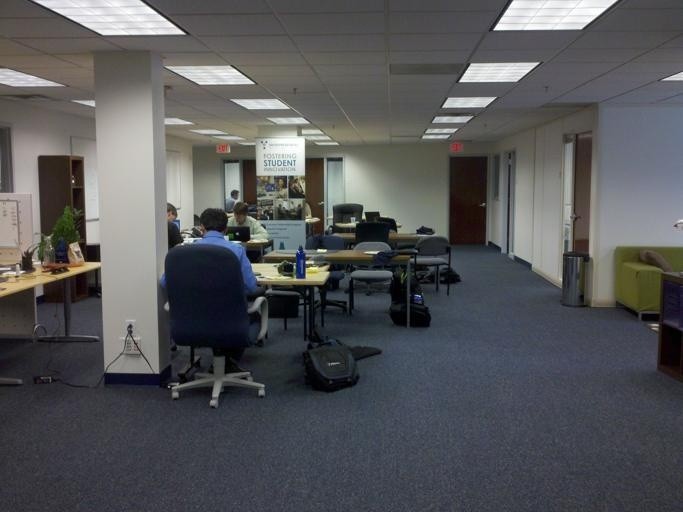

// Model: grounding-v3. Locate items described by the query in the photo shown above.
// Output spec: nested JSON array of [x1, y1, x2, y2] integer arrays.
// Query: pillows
[[639, 250, 673, 272]]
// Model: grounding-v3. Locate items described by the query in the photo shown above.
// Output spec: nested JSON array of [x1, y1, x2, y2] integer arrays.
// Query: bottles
[[295, 244, 306, 279], [43, 236, 56, 265], [15, 263, 21, 276]]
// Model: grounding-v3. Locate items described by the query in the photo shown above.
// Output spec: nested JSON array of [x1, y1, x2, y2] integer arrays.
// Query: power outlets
[[120, 337, 141, 355], [125, 319, 135, 335]]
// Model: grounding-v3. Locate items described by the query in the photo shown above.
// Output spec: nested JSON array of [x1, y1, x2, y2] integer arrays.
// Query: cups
[[350, 216, 355, 224]]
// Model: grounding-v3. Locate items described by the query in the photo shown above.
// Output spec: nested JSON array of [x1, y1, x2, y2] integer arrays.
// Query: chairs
[[162, 244, 269, 408], [304, 203, 452, 328]]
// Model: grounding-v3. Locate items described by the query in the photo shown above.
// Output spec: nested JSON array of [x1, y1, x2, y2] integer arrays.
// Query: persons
[[278, 178, 288, 198], [226, 202, 270, 240], [167, 203, 184, 249], [226, 190, 239, 212], [160, 208, 262, 347]]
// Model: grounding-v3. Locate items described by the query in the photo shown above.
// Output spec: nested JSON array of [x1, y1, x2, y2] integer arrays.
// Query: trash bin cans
[[562, 253, 590, 306]]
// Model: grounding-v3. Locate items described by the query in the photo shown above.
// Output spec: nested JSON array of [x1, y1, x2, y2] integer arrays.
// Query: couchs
[[615, 246, 683, 321]]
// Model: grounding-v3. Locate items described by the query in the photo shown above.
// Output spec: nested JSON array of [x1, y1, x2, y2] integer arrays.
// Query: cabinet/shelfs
[[656, 271, 683, 383], [38, 154, 89, 303]]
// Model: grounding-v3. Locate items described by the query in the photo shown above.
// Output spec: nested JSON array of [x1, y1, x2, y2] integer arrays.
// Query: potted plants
[[51, 205, 84, 264]]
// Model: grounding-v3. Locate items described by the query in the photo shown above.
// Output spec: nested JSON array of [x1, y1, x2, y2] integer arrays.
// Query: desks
[[250, 263, 330, 350], [0, 261, 102, 386], [176, 237, 274, 263], [261, 249, 411, 329]]
